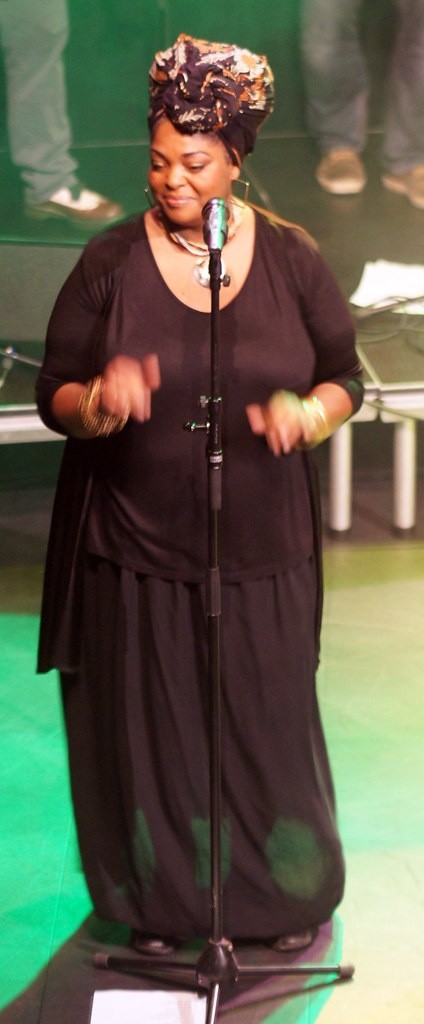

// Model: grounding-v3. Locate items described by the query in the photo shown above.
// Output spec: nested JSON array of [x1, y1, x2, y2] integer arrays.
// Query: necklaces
[[161, 196, 241, 288]]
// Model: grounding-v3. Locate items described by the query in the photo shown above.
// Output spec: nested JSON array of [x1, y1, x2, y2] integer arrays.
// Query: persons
[[33, 33, 365, 954], [0, 0, 124, 229], [299, 0, 424, 208]]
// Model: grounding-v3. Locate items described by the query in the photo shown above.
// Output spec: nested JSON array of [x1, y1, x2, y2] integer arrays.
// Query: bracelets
[[301, 397, 328, 451], [78, 377, 130, 437]]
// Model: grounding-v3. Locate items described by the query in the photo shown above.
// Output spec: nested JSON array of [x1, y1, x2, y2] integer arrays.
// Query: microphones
[[200, 196, 230, 253]]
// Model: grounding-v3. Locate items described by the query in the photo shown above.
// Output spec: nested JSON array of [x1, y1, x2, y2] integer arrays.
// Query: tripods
[[85, 250, 352, 1024]]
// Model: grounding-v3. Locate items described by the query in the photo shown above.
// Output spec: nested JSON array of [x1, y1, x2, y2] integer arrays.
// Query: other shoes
[[136, 932, 173, 954], [382, 164, 424, 208], [27, 186, 126, 232], [315, 149, 368, 197], [268, 929, 312, 951]]
